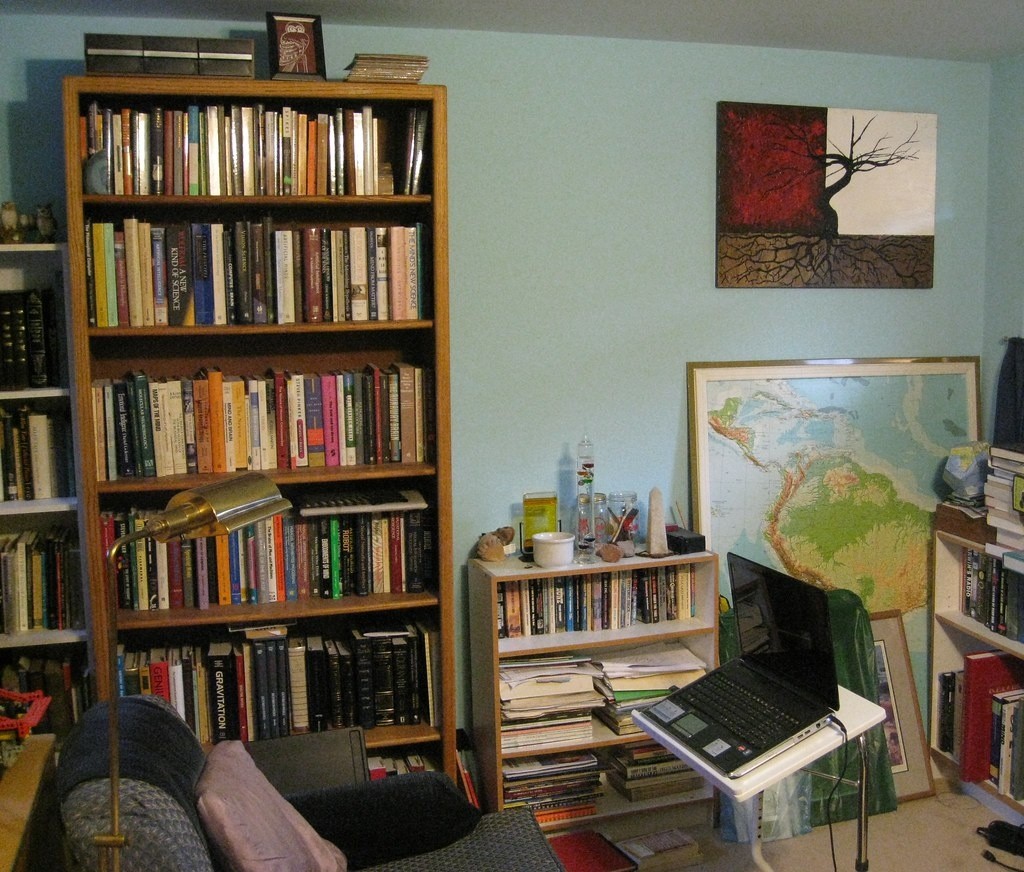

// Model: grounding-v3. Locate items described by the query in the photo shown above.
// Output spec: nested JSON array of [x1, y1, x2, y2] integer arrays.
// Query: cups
[[532, 533, 575, 567]]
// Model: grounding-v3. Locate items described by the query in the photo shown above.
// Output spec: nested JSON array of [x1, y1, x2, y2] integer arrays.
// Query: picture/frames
[[866, 609, 938, 804]]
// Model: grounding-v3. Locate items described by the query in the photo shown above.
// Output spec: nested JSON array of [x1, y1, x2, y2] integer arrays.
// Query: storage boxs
[[83, 32, 256, 79]]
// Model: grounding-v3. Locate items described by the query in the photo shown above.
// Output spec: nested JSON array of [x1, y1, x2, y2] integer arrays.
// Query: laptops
[[641, 551, 840, 779]]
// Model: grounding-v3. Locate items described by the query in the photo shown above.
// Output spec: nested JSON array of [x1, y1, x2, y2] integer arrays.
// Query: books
[[103, 493, 435, 612], [547, 824, 704, 872], [2, 652, 90, 768], [372, 755, 440, 781], [82, 96, 432, 201], [997, 528, 1024, 553], [112, 627, 439, 744], [962, 541, 1024, 643], [1, 530, 87, 639], [936, 650, 1023, 800], [496, 562, 699, 637], [981, 434, 1024, 535], [89, 363, 426, 484], [3, 403, 69, 503], [498, 641, 705, 824], [82, 221, 432, 327]]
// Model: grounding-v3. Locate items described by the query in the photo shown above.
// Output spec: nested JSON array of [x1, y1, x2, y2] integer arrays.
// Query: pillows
[[283, 769, 482, 870], [196, 738, 347, 872]]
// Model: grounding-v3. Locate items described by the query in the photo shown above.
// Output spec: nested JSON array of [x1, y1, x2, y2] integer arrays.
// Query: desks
[[0, 733, 59, 871], [635, 686, 885, 872]]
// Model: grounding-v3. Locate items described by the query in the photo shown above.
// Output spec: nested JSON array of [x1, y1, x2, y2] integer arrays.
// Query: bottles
[[593, 492, 607, 545], [609, 491, 639, 542], [572, 494, 591, 544]]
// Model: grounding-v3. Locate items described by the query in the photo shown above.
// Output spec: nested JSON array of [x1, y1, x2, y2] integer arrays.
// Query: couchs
[[57, 695, 564, 872]]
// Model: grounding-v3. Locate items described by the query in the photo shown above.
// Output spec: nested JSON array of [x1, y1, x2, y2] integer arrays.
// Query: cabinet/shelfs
[[685, 354, 983, 794], [926, 528, 1024, 817], [0, 243, 87, 653], [63, 77, 458, 786], [468, 552, 722, 833]]
